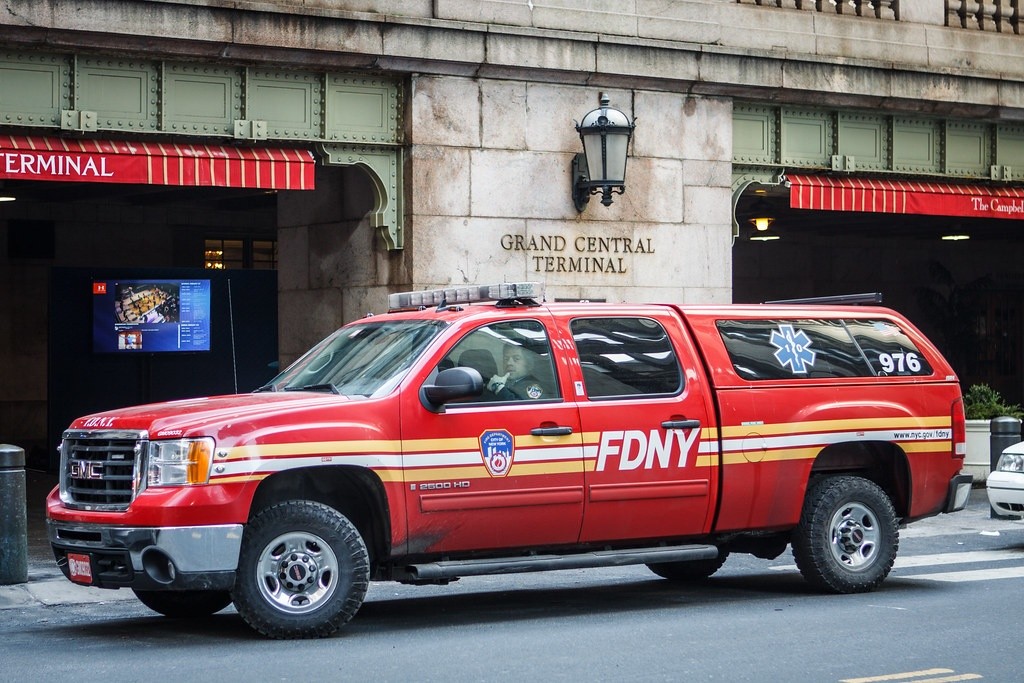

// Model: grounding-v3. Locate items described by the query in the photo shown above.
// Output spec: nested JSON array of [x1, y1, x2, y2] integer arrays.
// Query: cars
[[985, 441, 1024, 521]]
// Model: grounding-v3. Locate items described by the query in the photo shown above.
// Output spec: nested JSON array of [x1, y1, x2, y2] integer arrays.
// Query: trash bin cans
[[989, 417, 1022, 474], [0, 442, 30, 588]]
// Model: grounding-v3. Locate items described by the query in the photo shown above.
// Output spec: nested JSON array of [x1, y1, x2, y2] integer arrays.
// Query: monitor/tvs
[[88, 274, 215, 358]]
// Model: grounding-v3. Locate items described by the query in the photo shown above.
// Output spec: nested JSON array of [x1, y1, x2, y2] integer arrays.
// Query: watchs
[[491, 382, 503, 393]]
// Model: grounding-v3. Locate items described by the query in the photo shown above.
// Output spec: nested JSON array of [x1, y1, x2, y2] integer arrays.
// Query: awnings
[[0, 135, 316, 191], [785, 173, 1024, 219]]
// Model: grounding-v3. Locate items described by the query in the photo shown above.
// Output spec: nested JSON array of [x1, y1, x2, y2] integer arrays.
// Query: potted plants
[[958, 382, 1023, 483]]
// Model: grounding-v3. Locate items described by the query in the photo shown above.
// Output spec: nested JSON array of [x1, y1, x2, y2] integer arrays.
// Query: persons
[[933, 264, 979, 362], [486, 337, 555, 402]]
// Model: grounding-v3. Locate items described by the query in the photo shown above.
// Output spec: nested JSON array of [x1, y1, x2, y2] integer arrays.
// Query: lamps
[[572, 93, 638, 213]]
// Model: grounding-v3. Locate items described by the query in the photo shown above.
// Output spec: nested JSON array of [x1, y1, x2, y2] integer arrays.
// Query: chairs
[[457, 349, 498, 383]]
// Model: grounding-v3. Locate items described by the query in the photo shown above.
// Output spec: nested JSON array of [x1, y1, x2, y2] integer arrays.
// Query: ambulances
[[44, 282, 975, 640]]
